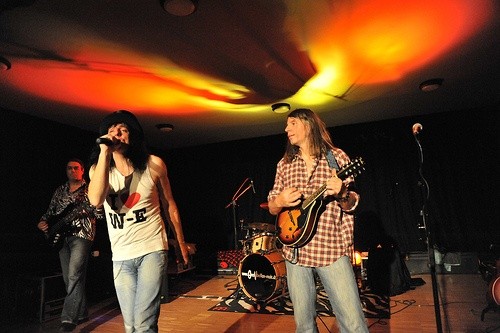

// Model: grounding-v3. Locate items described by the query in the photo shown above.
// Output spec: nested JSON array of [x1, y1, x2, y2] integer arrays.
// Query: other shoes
[[77, 317, 89, 325], [58, 323, 76, 333]]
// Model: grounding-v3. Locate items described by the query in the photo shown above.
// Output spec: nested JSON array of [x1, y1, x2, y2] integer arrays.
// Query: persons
[[38, 158, 105, 333], [267, 109, 370, 333], [87, 109, 189, 333]]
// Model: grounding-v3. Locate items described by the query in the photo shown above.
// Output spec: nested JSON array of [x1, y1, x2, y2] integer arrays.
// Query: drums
[[237, 248, 287, 302], [237, 223, 278, 254]]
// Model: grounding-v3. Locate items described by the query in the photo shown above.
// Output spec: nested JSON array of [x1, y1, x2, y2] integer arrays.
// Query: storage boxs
[[11, 273, 66, 321]]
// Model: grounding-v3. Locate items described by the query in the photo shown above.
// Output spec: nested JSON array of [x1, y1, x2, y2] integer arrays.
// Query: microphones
[[249, 180, 256, 193], [96, 138, 118, 147], [412, 123, 423, 135]]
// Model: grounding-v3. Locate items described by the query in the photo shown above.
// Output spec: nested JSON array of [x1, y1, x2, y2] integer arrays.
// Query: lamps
[[419, 79, 443, 92], [272, 103, 291, 113]]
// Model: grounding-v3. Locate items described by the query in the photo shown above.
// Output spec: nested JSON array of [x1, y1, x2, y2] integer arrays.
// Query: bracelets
[[179, 240, 186, 244], [338, 187, 350, 203]]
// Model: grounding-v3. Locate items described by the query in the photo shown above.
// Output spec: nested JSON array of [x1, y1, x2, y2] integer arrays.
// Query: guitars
[[40, 201, 89, 251], [274, 155, 366, 248]]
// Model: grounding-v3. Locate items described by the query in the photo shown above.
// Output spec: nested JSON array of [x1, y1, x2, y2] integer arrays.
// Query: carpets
[[209, 288, 391, 320]]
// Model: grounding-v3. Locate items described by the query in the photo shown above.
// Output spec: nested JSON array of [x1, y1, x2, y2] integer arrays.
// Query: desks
[[367, 248, 426, 295]]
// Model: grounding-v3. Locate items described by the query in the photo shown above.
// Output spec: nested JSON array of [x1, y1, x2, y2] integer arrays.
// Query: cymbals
[[259, 202, 269, 210]]
